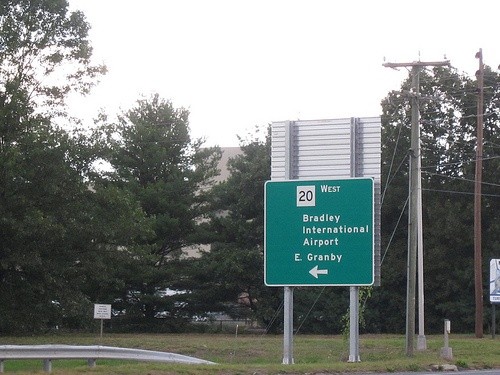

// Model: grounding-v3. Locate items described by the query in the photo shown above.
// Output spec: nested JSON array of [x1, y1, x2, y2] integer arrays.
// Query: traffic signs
[[262, 178, 375, 286]]
